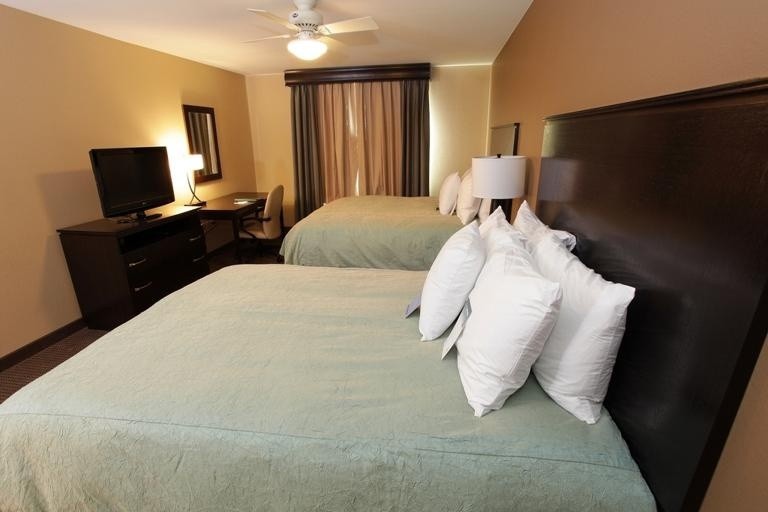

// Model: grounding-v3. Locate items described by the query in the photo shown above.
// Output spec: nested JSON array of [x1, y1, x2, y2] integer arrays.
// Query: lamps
[[470, 152, 526, 224], [286, 38, 326, 61], [182, 154, 207, 206]]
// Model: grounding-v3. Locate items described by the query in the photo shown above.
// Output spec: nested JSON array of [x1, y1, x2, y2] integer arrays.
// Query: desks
[[193, 190, 283, 264]]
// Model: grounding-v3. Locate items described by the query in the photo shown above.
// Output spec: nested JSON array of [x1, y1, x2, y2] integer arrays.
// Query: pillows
[[437, 170, 461, 215], [418, 219, 487, 341], [477, 197, 491, 224], [454, 206, 561, 425], [456, 166, 482, 226], [508, 197, 640, 425]]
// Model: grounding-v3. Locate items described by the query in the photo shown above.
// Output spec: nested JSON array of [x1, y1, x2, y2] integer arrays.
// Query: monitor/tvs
[[88, 146, 175, 223]]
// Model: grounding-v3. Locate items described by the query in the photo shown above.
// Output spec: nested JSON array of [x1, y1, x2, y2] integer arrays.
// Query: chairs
[[239, 184, 284, 264]]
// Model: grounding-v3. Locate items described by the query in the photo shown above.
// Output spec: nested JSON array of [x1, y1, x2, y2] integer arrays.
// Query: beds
[[1, 76, 767, 512], [277, 121, 520, 271]]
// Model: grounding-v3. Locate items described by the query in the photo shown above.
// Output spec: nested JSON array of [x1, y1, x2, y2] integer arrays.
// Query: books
[[234, 195, 267, 202]]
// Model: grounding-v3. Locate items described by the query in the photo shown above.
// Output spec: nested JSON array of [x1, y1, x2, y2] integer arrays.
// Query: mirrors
[[181, 103, 222, 183]]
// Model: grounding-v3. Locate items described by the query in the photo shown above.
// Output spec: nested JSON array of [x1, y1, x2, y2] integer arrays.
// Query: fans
[[237, 0, 380, 49]]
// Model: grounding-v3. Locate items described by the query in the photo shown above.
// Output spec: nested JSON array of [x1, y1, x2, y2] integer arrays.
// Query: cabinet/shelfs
[[55, 205, 211, 332]]
